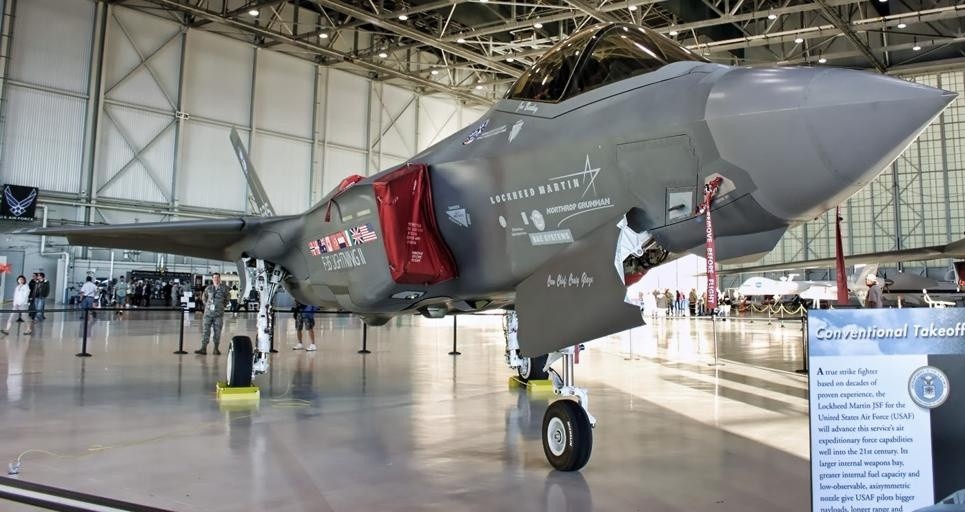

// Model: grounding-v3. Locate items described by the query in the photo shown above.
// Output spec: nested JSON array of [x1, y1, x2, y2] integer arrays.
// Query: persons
[[229, 285, 239, 311], [665, 289, 697, 315], [293, 303, 320, 350], [194, 273, 231, 355], [113, 275, 192, 315], [864, 274, 883, 308], [80, 276, 97, 319], [200, 281, 210, 313], [93, 282, 101, 313], [249, 286, 257, 301], [28, 273, 39, 316], [0, 276, 32, 335], [33, 272, 50, 323]]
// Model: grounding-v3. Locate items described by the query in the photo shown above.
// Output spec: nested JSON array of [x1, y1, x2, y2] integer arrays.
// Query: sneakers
[[292, 342, 317, 352], [1, 328, 33, 336], [192, 349, 222, 355]]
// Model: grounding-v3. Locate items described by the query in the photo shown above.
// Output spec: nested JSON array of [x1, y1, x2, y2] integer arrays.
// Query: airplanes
[[6, 20, 963, 474], [691, 230, 965, 308]]
